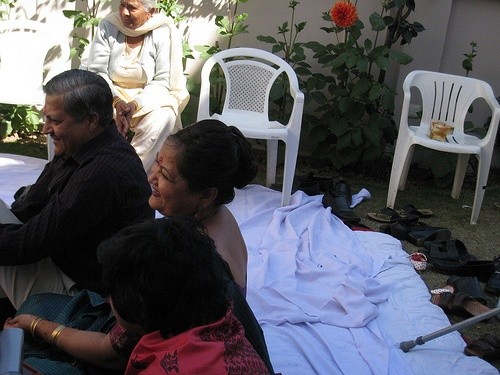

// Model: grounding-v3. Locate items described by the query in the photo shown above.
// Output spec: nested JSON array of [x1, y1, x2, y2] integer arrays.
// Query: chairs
[[195, 47, 304, 207], [386, 70, 500, 226], [0, 19, 72, 162]]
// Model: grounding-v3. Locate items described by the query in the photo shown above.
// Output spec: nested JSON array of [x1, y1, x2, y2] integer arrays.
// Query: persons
[[0, 67, 156, 311], [4, 118, 247, 375], [97, 216, 270, 375], [88, 0, 190, 174]]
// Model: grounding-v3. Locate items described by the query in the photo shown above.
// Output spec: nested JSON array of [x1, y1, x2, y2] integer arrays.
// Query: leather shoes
[[271, 172, 332, 196], [321, 179, 361, 224]]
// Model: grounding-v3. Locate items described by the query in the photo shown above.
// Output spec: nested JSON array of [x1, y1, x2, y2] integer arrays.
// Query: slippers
[[367, 204, 451, 247], [418, 238, 500, 363]]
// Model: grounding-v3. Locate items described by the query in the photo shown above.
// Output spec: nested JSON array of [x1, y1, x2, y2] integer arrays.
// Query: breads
[[429, 121, 451, 141]]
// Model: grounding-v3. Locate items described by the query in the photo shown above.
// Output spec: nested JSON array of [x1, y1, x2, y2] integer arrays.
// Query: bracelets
[[131, 100, 139, 112], [47, 324, 66, 347], [30, 316, 46, 336], [112, 98, 122, 108]]
